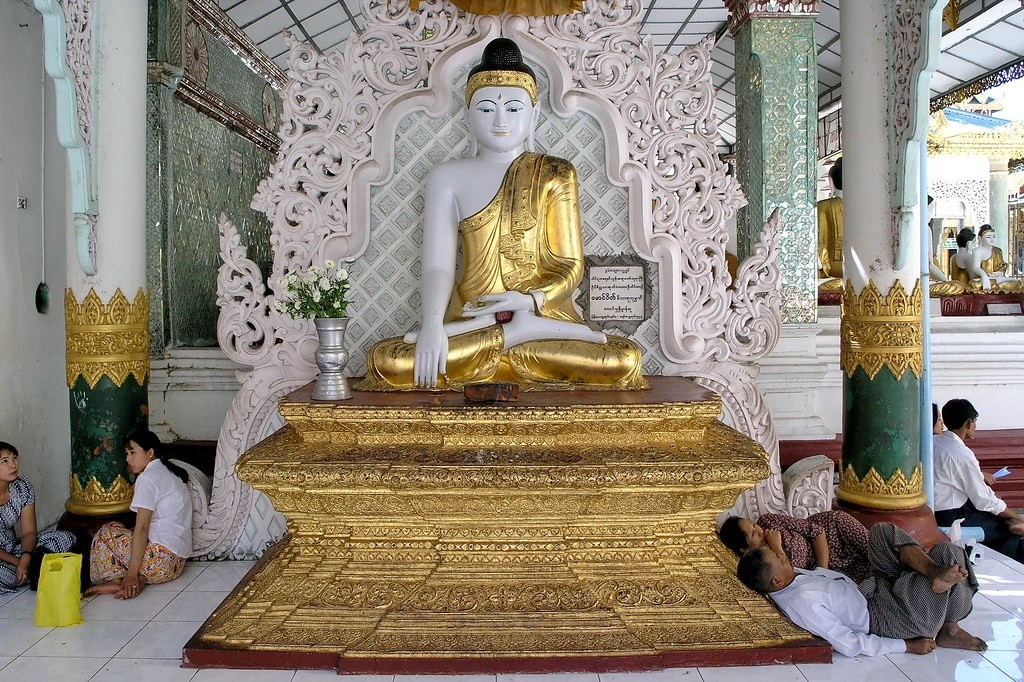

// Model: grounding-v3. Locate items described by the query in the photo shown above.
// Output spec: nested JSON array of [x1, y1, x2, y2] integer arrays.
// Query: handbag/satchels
[[35, 552, 85, 627], [30, 545, 88, 593]]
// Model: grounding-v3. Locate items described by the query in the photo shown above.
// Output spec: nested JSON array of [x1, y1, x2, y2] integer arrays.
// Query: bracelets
[[21, 550, 32, 556]]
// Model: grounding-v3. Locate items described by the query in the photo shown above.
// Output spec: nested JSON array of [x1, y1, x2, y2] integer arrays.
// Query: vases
[[314, 317, 353, 401]]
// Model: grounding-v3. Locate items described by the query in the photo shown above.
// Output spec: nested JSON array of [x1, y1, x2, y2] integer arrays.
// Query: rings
[[133, 586, 136, 588]]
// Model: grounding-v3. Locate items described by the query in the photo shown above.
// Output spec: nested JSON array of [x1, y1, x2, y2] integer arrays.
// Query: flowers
[[274, 257, 355, 321]]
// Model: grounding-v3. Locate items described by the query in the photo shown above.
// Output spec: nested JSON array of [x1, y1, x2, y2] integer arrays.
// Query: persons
[[82, 429, 193, 599], [736, 521, 988, 656], [719, 510, 870, 585], [352, 38, 653, 391], [932, 399, 1024, 563], [970, 224, 1022, 291], [949, 228, 976, 283], [817, 158, 844, 294], [0, 441, 76, 597], [927, 195, 964, 295]]
